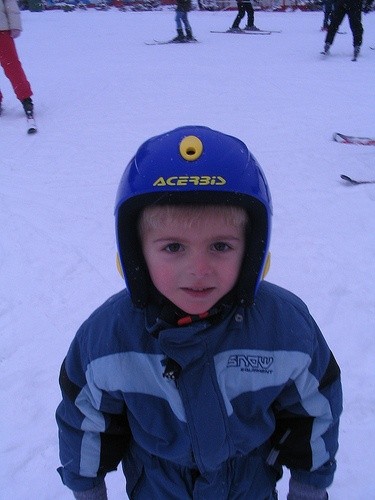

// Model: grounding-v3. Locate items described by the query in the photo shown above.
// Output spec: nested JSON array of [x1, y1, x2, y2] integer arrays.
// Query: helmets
[[112, 126, 272, 307]]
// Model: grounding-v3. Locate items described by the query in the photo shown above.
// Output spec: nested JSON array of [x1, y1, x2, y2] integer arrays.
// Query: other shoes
[[325, 42, 331, 51], [246, 25, 259, 31], [23, 99, 34, 115], [185, 34, 194, 41], [174, 34, 185, 41], [354, 46, 359, 56], [230, 27, 243, 32]]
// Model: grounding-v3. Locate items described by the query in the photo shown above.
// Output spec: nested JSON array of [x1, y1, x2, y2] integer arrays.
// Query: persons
[[172, 0, 196, 42], [55, 127, 343, 500], [231, 0, 260, 32], [321, 0, 372, 59], [0, 0, 34, 120]]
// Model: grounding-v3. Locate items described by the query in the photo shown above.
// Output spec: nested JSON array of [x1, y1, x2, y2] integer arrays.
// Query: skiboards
[[332, 131, 375, 184], [210, 23, 283, 36], [0, 98, 38, 135], [320, 41, 360, 61], [320, 26, 348, 35], [144, 32, 204, 44]]
